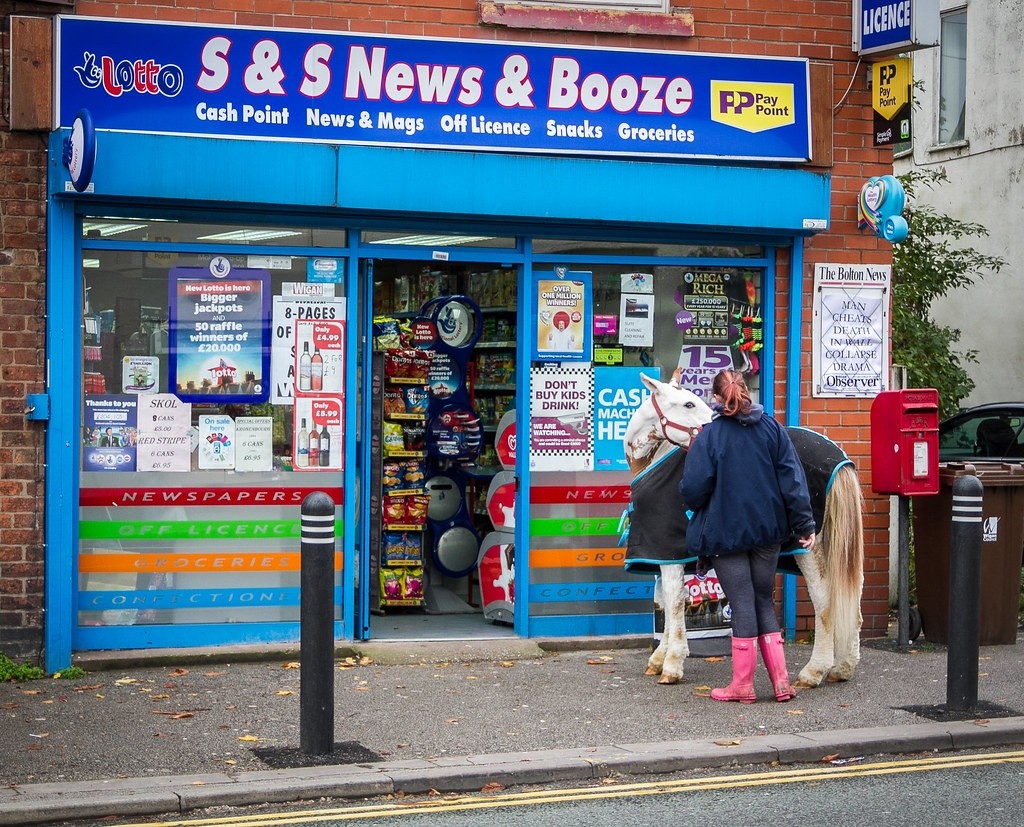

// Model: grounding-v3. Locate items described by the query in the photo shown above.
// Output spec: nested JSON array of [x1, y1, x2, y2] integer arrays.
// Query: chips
[[410, 509, 425, 517], [383, 476, 401, 486], [388, 510, 404, 518], [406, 472, 423, 481]]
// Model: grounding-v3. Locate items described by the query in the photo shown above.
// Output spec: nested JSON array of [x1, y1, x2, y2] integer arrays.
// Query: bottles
[[297, 418, 309, 467], [311, 345, 323, 391], [319, 425, 330, 465], [300, 342, 311, 390], [308, 420, 320, 467]]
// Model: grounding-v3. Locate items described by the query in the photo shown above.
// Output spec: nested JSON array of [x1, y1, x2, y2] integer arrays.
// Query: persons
[[101, 427, 120, 448], [680, 370, 817, 704], [548, 321, 575, 351]]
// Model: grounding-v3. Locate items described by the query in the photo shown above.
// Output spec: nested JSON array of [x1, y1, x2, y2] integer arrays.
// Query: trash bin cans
[[913, 460, 1024, 647]]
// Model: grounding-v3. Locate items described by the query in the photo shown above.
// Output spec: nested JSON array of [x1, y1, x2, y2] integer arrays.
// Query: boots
[[758, 632, 796, 701], [710, 636, 758, 704]]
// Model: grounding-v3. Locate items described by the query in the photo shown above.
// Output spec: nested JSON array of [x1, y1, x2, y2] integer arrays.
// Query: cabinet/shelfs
[[370, 270, 516, 614]]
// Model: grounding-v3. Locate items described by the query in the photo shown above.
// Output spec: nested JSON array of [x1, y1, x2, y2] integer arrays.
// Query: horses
[[620, 368, 864, 690]]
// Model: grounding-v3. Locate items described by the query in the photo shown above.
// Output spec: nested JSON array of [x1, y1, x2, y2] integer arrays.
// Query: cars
[[936, 401, 1024, 462]]
